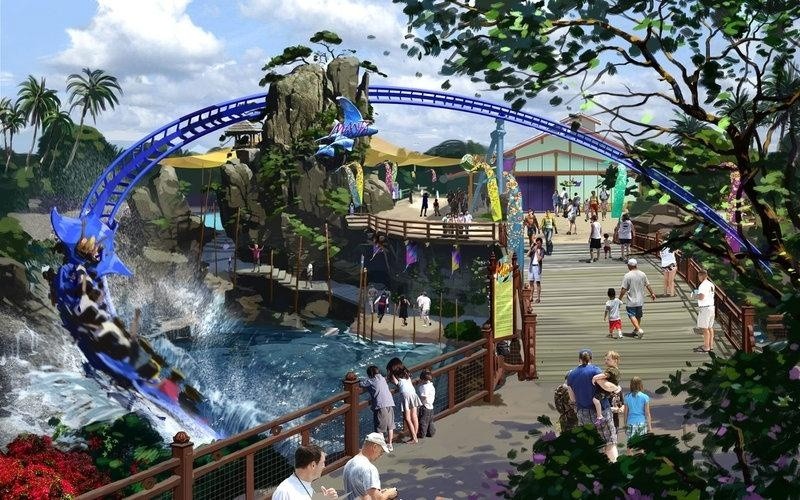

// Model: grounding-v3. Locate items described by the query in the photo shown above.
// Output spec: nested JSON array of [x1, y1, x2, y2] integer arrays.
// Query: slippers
[[694, 345, 713, 353]]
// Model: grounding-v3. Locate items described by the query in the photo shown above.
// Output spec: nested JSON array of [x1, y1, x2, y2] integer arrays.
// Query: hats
[[579, 349, 592, 358], [364, 432, 390, 454], [627, 259, 638, 266]]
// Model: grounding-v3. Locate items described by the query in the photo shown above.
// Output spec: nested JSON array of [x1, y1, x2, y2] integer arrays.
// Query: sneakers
[[594, 417, 605, 427], [634, 329, 644, 338]]
